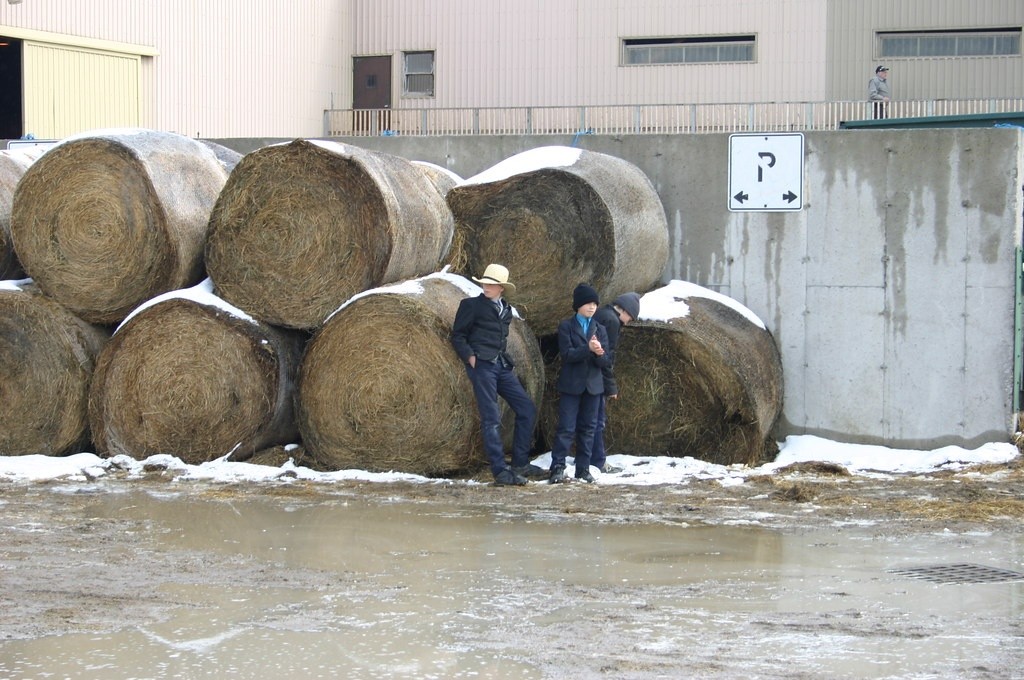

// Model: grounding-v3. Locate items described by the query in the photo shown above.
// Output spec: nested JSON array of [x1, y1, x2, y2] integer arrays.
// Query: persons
[[550, 282, 609, 485], [574, 292, 641, 473], [451, 264, 550, 488], [868, 65, 891, 120]]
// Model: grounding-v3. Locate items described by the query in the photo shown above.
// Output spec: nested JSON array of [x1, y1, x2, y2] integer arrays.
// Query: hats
[[572, 283, 599, 312], [471, 263, 516, 297], [613, 292, 640, 323]]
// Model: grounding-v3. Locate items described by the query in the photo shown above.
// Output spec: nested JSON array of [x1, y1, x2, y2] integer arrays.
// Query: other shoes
[[600, 462, 624, 474], [550, 465, 566, 483], [512, 463, 548, 480], [494, 467, 529, 486], [576, 469, 595, 483]]
